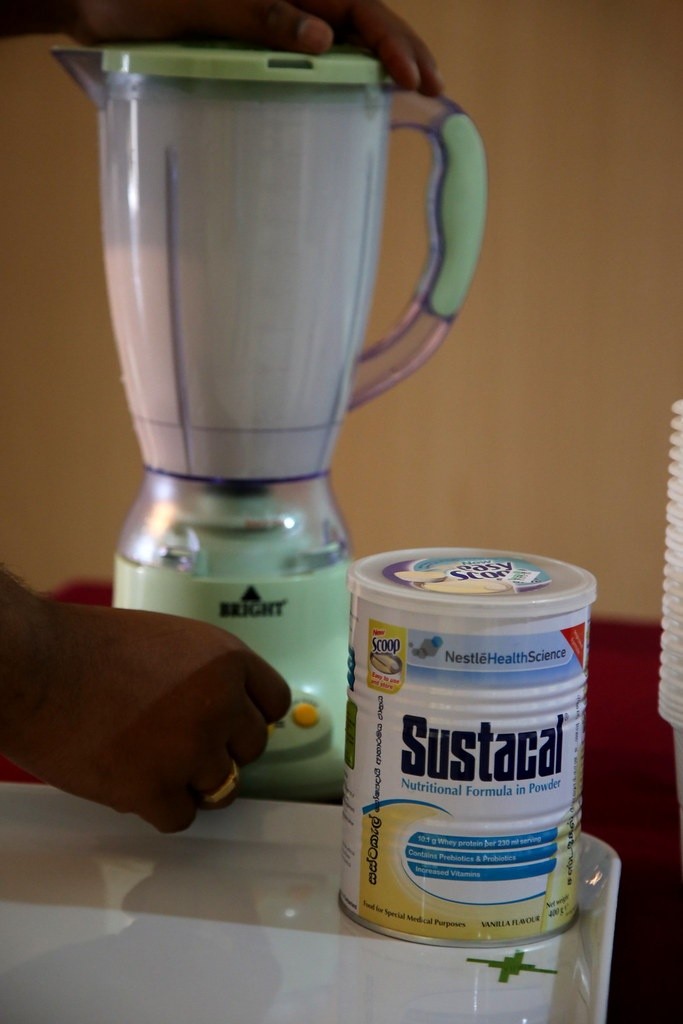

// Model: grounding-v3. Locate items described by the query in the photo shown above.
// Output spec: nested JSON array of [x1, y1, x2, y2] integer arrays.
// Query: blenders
[[42, 42, 492, 796]]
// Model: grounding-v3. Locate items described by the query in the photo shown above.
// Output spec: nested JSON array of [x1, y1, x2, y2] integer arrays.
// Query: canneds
[[339, 547, 597, 947]]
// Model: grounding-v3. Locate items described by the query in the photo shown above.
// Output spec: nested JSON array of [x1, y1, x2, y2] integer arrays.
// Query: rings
[[203, 759, 240, 803]]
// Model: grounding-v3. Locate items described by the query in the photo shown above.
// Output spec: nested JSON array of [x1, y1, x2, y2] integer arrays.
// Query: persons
[[0, 1, 443, 838]]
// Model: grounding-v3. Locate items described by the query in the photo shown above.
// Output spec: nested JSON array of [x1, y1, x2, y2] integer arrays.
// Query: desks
[[0, 577, 683, 1024]]
